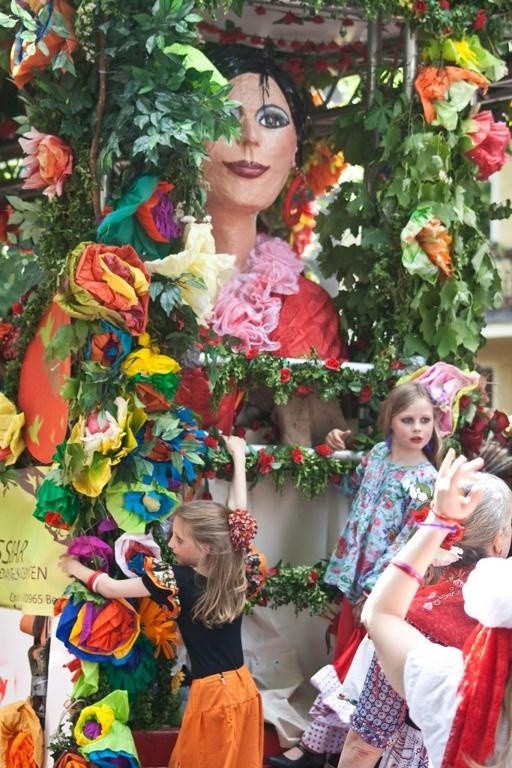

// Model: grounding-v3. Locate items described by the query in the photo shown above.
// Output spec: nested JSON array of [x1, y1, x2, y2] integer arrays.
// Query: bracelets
[[389, 557, 425, 587], [416, 518, 459, 534], [88, 568, 108, 595], [428, 499, 470, 524]]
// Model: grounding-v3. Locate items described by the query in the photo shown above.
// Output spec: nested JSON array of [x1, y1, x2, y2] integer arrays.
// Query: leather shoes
[[265, 741, 326, 768]]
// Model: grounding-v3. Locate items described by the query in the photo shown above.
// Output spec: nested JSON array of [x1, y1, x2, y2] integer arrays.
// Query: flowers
[[0, 0, 512, 768]]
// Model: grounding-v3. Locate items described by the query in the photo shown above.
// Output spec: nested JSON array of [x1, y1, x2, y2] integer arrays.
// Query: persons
[[115, 38, 347, 368], [338, 468, 512, 767], [265, 377, 441, 767], [57, 432, 266, 768], [358, 445, 511, 767]]
